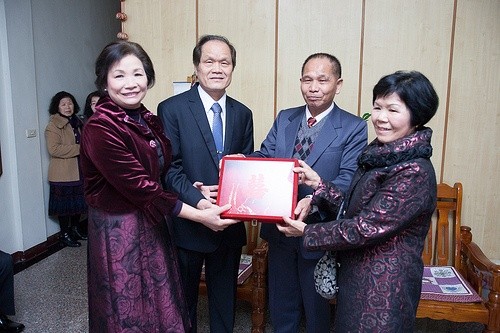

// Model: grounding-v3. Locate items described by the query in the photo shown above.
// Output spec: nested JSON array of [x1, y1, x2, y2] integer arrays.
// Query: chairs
[[416, 182, 500, 333], [198, 220, 268, 333]]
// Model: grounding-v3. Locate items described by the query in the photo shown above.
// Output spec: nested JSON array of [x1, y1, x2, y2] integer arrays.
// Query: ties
[[210, 102, 223, 160], [308, 117, 317, 128]]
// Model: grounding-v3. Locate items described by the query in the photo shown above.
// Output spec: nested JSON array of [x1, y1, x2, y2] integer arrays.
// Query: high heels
[[72, 227, 88, 241], [58, 231, 81, 247]]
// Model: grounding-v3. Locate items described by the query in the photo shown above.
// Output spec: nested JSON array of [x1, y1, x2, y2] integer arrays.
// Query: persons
[[0, 248, 26, 333], [83, 90, 105, 122], [78, 39, 243, 333], [276, 70, 439, 333], [218, 53, 368, 333], [44, 90, 89, 247], [155, 35, 255, 333]]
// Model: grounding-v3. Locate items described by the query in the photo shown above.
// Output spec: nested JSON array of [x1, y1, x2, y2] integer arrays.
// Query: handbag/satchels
[[314, 197, 345, 299]]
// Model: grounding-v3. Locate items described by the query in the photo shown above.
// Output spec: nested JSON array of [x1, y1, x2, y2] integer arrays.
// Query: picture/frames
[[216, 156, 301, 224]]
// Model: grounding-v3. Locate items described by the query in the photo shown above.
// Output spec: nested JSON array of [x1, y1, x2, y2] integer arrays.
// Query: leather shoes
[[0, 318, 25, 333]]
